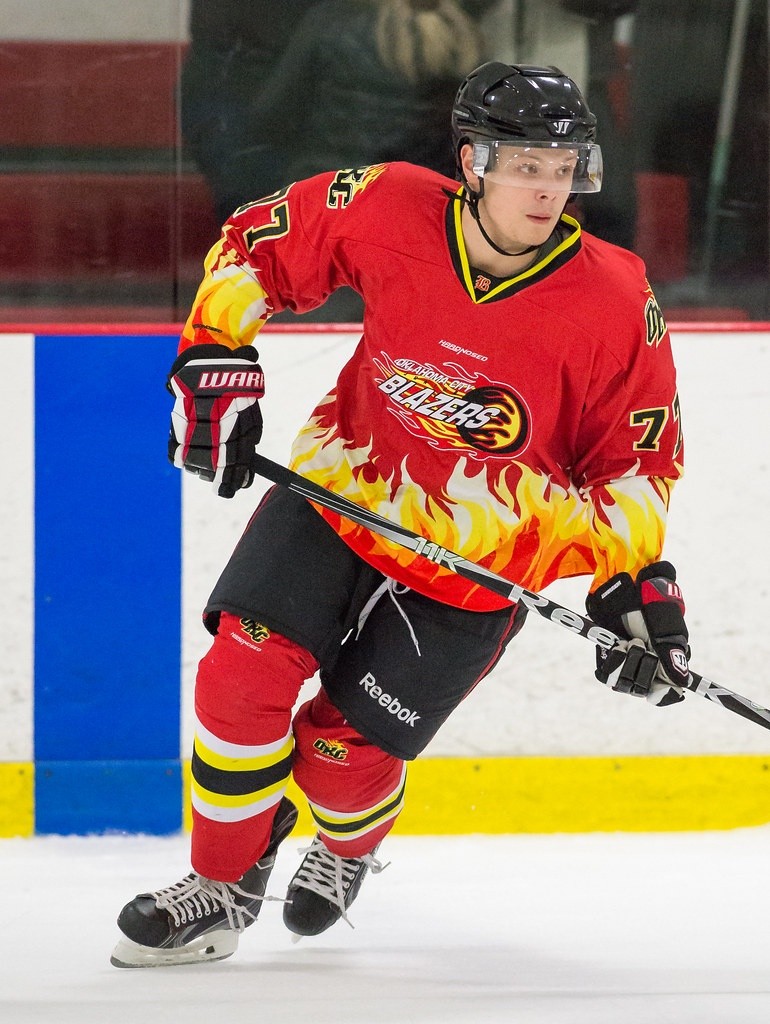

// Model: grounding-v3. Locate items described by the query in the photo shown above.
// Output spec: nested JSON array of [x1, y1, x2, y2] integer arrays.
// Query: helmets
[[454, 60, 604, 193]]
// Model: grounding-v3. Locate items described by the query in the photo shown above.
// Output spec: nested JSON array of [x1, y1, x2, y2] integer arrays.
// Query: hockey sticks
[[250, 452, 769, 731]]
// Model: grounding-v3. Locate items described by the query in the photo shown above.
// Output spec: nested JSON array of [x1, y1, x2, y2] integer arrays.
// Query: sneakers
[[108, 799, 296, 964], [285, 836, 380, 934]]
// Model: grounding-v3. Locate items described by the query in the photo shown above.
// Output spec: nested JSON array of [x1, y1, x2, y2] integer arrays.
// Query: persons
[[111, 61, 692, 969], [182, 0, 729, 290]]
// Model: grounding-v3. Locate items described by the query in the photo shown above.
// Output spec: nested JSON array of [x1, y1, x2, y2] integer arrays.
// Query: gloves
[[166, 344, 265, 497], [584, 562, 692, 707]]
